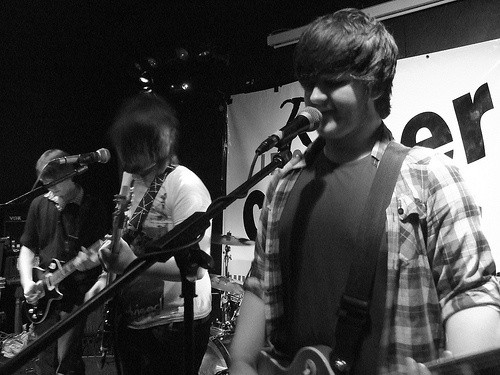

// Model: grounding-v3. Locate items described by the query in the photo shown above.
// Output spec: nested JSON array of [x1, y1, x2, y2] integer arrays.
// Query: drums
[[196, 334, 237, 375]]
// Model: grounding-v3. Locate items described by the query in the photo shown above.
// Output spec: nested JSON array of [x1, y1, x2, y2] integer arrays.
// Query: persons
[[17, 149, 109, 375], [84, 94, 213, 375], [229, 8, 500, 375]]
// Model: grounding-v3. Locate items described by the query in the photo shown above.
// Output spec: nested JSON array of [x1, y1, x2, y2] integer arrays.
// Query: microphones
[[256, 105, 323, 155], [50, 148, 111, 165]]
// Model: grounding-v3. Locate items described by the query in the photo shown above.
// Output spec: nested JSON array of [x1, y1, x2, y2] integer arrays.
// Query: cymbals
[[209, 274, 245, 294], [211, 236, 249, 246]]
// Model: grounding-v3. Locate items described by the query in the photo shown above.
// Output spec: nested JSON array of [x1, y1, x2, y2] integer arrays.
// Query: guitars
[[254, 345, 348, 375], [90, 184, 139, 371], [26, 238, 105, 324]]
